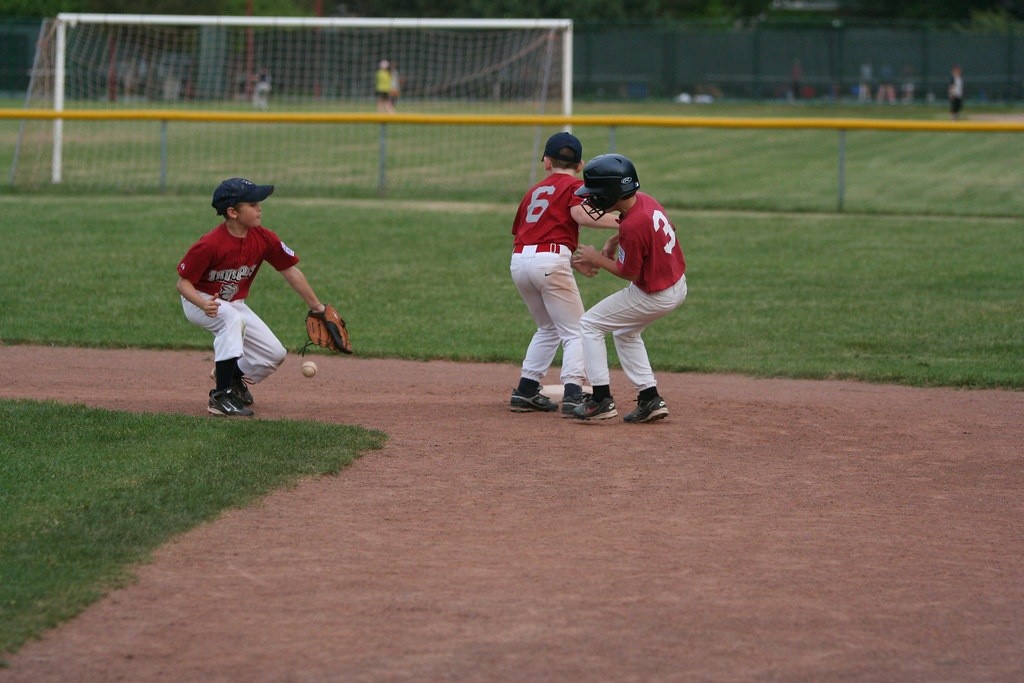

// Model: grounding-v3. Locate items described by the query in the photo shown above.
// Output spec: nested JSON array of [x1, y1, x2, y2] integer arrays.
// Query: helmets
[[574, 154, 640, 209]]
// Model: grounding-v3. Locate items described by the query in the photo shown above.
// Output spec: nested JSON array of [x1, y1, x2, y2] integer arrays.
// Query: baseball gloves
[[304, 303, 354, 356]]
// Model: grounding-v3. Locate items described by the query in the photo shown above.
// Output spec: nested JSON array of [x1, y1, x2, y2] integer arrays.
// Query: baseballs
[[301, 361, 318, 378]]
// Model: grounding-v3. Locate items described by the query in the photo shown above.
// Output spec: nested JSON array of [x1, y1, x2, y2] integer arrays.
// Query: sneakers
[[208, 388, 254, 418], [509, 385, 558, 413], [623, 395, 669, 423], [572, 393, 618, 421], [560, 393, 586, 418], [211, 368, 253, 404]]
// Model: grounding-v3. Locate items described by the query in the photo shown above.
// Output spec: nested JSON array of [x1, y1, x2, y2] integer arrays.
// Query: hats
[[212, 177, 275, 213], [541, 132, 582, 163]]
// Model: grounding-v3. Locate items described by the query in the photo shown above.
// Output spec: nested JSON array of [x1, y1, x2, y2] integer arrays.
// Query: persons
[[509, 131, 689, 423], [175, 176, 351, 418], [376, 59, 400, 111], [945, 63, 962, 117], [791, 58, 917, 104], [255, 73, 272, 111]]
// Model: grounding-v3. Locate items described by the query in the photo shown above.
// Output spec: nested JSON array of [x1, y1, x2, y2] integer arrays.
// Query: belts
[[513, 244, 560, 254]]
